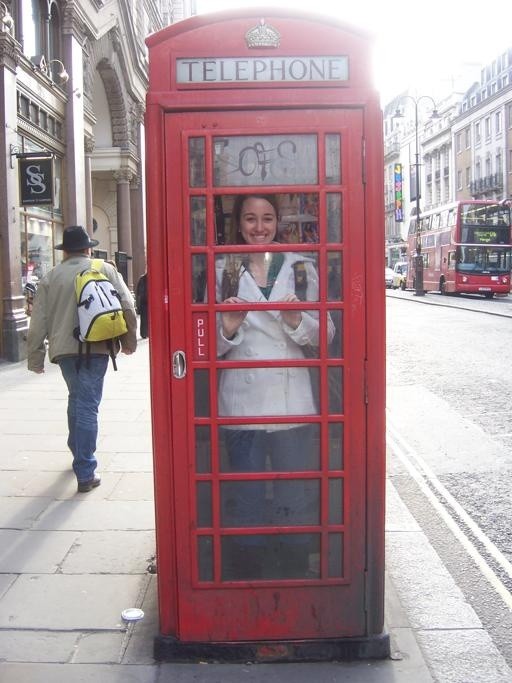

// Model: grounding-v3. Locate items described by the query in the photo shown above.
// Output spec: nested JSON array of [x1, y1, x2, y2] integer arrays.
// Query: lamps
[[42, 60, 69, 82]]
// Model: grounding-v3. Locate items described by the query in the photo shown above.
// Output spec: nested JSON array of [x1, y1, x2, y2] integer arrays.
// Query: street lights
[[391, 96, 442, 297]]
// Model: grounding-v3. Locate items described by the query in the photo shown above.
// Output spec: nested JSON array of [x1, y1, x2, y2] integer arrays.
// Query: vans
[[392, 261, 408, 289]]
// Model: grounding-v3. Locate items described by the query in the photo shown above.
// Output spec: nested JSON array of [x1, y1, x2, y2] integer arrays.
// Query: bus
[[404, 198, 512, 299]]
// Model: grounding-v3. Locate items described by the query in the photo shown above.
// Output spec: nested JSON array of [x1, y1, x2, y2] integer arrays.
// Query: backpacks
[[73, 258, 129, 342]]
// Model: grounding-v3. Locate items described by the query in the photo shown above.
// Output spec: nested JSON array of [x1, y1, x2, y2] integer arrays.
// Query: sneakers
[[78, 475, 101, 492]]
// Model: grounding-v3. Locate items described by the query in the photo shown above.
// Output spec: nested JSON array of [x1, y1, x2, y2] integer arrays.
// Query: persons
[[136, 264, 148, 338], [25, 224, 137, 493], [201, 194, 336, 582]]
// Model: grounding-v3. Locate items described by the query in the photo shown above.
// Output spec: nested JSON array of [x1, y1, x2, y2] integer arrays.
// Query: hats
[[54, 225, 99, 252]]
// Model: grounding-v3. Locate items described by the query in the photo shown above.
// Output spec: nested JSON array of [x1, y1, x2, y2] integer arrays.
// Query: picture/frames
[[18, 156, 56, 208]]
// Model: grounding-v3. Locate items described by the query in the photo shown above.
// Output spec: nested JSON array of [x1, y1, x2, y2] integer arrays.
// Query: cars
[[385, 267, 394, 289]]
